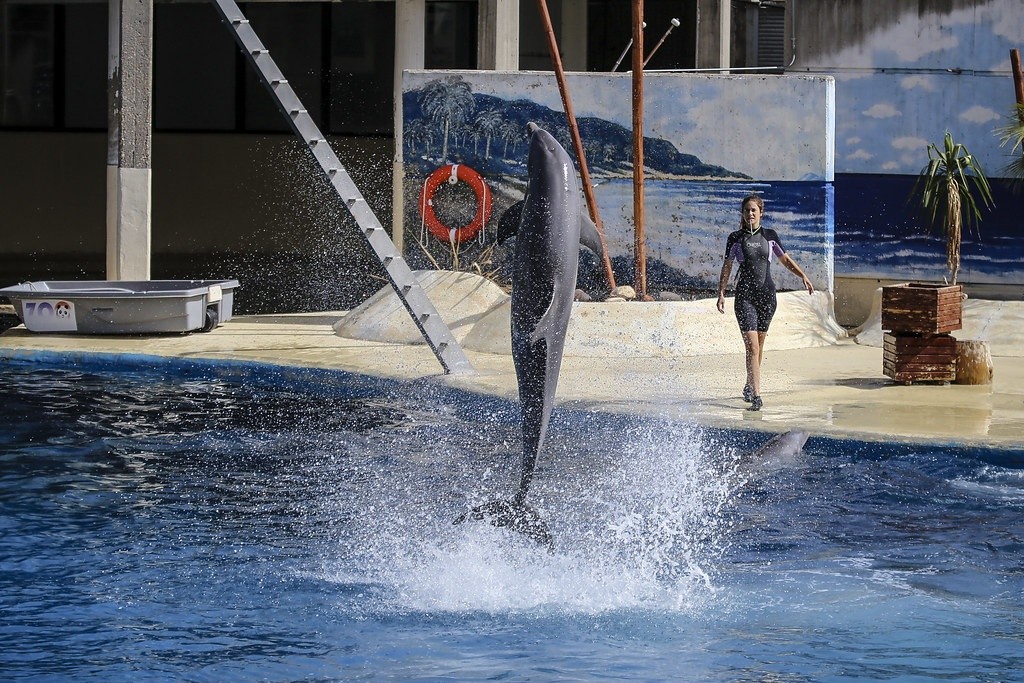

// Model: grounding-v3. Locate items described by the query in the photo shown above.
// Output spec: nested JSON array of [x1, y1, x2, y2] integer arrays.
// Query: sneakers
[[743, 384, 752, 401], [751, 395, 762, 408]]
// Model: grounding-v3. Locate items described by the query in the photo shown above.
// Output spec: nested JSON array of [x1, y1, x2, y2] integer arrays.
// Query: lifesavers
[[419, 164, 492, 246]]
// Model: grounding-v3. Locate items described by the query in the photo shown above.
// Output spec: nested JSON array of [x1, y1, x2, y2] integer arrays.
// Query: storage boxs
[[880, 280, 967, 335], [880, 329, 958, 386]]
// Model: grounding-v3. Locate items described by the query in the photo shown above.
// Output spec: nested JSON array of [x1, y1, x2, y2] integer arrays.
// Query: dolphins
[[451, 122, 603, 556], [736, 430, 810, 455]]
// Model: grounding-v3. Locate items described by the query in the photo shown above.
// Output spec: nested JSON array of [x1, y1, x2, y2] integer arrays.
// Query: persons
[[717, 194, 814, 411]]
